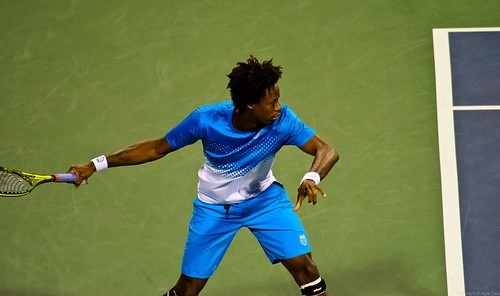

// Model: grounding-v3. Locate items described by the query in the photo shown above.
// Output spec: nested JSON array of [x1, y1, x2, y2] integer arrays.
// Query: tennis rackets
[[0, 165, 89, 198]]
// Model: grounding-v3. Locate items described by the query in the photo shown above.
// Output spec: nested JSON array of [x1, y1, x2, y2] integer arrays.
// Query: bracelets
[[299, 172, 320, 186], [91, 155, 108, 172]]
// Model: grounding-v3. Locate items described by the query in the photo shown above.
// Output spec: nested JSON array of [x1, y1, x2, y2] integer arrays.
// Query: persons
[[65, 54, 340, 296]]
